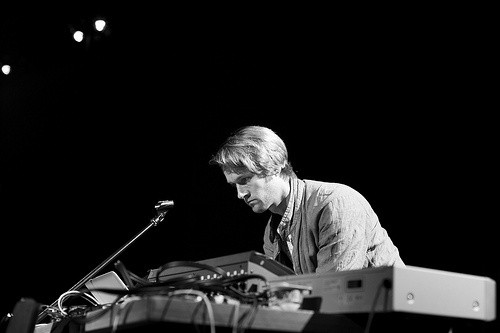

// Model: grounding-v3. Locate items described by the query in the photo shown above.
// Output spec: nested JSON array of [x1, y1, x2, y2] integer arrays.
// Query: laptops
[[85, 271, 130, 310]]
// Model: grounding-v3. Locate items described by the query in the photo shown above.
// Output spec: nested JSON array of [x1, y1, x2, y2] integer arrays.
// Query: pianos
[[142, 248, 497, 322]]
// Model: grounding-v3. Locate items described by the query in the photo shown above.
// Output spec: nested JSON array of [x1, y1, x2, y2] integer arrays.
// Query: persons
[[207, 125, 406, 275]]
